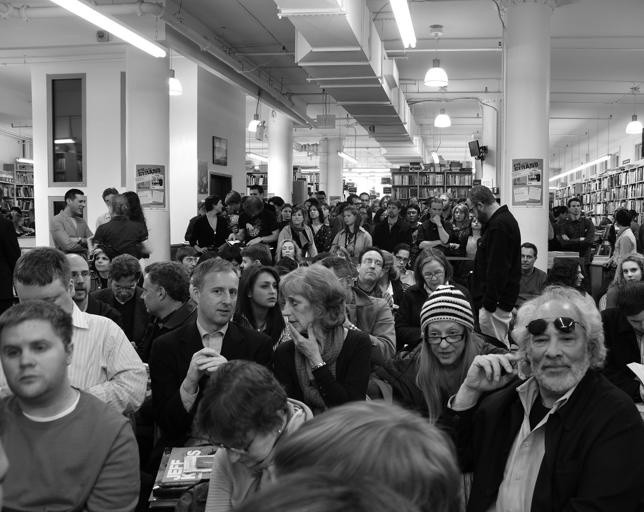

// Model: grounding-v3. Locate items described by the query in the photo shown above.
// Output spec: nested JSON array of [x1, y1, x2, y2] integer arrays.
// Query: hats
[[419, 285, 475, 334]]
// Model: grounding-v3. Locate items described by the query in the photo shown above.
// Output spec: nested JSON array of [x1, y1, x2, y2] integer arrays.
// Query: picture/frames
[[211, 135, 230, 168]]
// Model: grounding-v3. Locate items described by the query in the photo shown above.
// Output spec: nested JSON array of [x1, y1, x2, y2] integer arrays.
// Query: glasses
[[525, 315, 589, 336], [72, 272, 93, 281], [425, 329, 468, 345], [421, 271, 445, 280], [206, 430, 261, 457]]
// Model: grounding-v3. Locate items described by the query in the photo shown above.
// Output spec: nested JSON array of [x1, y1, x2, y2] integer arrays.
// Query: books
[[0, 163, 34, 218], [393, 172, 473, 200], [552, 166, 644, 227]]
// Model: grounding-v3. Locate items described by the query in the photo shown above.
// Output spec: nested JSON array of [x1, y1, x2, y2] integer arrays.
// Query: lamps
[[244, 127, 269, 163], [423, 22, 452, 91], [624, 86, 644, 136], [54, 115, 77, 145], [432, 85, 454, 129], [388, 0, 420, 52], [14, 140, 33, 164], [167, 48, 183, 99], [45, 0, 167, 62], [547, 117, 613, 185], [334, 113, 360, 165], [246, 91, 266, 133]]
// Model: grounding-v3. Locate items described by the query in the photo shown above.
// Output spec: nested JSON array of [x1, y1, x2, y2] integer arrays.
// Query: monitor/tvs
[[468, 140, 481, 156]]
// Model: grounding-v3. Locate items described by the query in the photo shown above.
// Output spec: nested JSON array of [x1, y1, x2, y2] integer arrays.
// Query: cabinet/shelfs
[[549, 165, 643, 230], [291, 180, 309, 207], [0, 181, 16, 214], [417, 171, 445, 204], [245, 173, 269, 204], [2, 162, 36, 231], [445, 171, 472, 203], [391, 171, 418, 204]]
